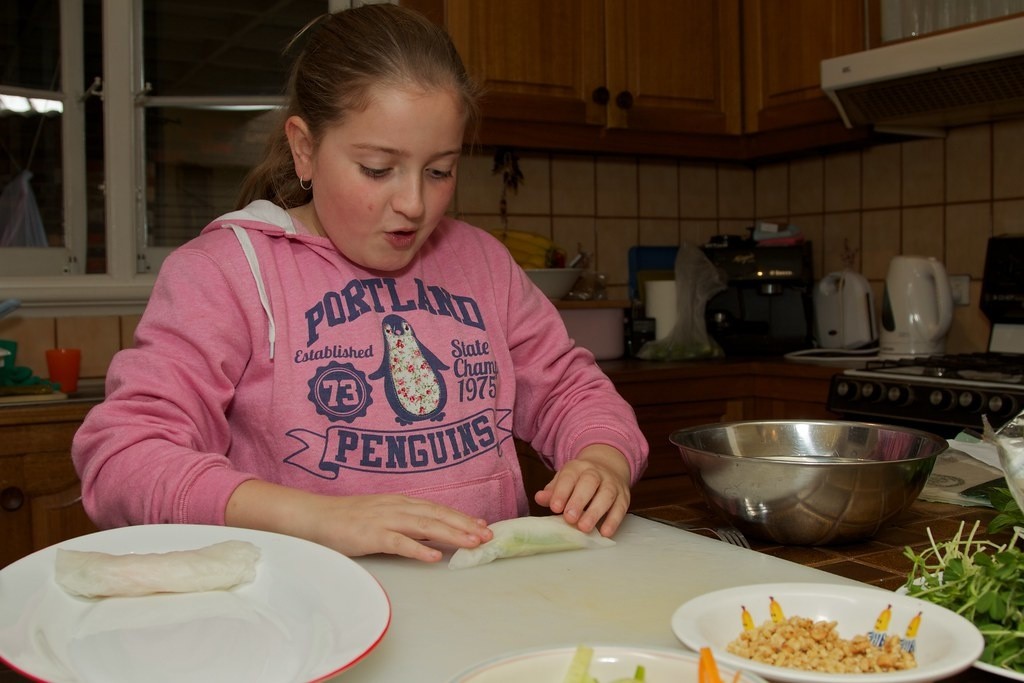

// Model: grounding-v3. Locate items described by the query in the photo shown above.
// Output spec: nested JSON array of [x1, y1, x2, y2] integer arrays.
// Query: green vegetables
[[900, 484, 1024, 673]]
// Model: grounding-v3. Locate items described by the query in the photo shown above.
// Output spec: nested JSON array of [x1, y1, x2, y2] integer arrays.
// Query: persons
[[70, 3, 650, 563]]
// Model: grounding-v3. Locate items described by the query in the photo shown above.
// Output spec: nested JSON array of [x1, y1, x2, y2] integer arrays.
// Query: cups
[[643, 279, 682, 339], [44, 347, 82, 394]]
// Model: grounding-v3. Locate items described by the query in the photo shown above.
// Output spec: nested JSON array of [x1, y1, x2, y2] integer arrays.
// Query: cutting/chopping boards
[[307, 511, 894, 683]]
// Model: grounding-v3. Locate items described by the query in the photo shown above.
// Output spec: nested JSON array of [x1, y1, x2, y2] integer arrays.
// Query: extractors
[[819, 1, 1024, 138]]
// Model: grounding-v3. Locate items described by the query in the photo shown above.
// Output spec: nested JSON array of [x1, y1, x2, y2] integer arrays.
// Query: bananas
[[488, 229, 564, 269]]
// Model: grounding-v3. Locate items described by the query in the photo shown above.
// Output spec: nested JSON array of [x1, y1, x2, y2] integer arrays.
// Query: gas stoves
[[828, 235, 1024, 436]]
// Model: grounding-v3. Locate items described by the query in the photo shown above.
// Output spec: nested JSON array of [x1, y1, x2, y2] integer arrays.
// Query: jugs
[[705, 279, 788, 349]]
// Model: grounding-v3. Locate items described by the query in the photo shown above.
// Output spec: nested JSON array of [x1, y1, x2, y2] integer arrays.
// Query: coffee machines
[[701, 237, 818, 354]]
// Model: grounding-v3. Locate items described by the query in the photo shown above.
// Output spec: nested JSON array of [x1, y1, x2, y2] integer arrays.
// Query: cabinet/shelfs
[[384, 0, 880, 163], [0, 398, 834, 573]]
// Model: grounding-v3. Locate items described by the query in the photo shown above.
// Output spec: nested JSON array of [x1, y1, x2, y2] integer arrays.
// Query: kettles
[[876, 254, 952, 361]]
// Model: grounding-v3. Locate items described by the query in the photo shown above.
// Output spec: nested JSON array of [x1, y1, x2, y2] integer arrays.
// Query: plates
[[897, 567, 1023, 681], [437, 643, 765, 682], [671, 583, 983, 683], [0, 522, 394, 683]]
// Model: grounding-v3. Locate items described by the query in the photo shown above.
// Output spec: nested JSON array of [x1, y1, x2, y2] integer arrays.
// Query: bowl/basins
[[672, 420, 949, 545], [519, 266, 578, 301]]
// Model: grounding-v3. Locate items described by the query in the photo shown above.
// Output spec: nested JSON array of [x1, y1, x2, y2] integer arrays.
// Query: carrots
[[697, 647, 743, 683]]
